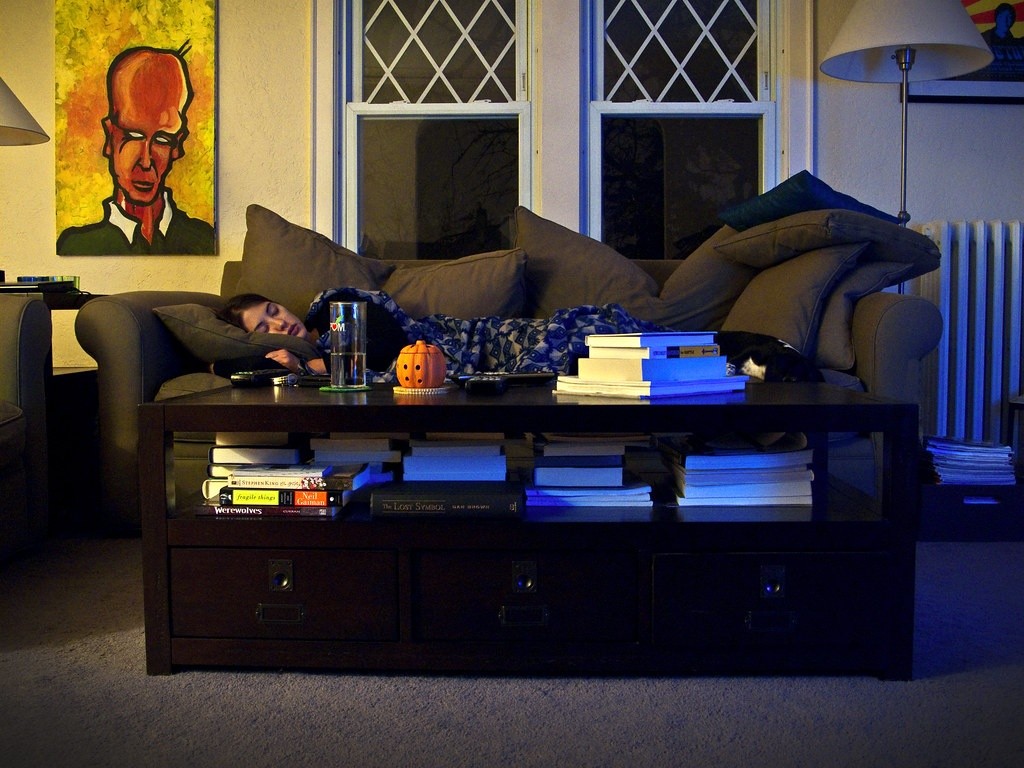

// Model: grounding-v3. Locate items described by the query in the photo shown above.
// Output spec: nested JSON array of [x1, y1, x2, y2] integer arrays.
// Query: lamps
[[0, 77, 51, 284], [821, 0, 995, 288]]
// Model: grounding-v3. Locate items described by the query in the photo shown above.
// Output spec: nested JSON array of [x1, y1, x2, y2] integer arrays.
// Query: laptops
[[0, 280, 75, 294]]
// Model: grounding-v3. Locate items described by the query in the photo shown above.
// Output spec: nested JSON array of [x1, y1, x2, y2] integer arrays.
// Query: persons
[[980, 3, 1017, 48], [217, 285, 737, 385]]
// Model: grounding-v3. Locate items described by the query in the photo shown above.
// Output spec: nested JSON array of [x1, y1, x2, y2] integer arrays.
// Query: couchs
[[0, 293, 59, 550], [75, 260, 941, 532]]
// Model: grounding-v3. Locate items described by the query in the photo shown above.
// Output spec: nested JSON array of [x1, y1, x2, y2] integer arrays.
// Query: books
[[203, 430, 816, 523], [924, 433, 1016, 486], [551, 331, 749, 400]]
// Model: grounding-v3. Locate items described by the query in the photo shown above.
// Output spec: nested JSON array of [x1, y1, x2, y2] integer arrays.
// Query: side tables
[[5, 293, 108, 537]]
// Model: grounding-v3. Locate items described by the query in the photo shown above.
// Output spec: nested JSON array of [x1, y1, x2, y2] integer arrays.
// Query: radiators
[[919, 222, 1024, 469]]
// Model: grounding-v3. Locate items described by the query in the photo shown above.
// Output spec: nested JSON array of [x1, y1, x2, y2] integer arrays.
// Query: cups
[[330, 301, 367, 388]]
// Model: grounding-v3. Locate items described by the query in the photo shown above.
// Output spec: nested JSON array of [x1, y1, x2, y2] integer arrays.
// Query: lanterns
[[396, 340, 447, 389]]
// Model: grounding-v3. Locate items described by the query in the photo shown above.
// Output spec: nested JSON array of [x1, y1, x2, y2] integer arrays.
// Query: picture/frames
[[901, 1, 1024, 103]]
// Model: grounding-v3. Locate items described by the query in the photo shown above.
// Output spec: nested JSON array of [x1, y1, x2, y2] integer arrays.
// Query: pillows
[[153, 169, 916, 369]]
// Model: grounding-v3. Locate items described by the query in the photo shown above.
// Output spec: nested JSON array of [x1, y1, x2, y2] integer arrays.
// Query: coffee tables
[[137, 375, 930, 679]]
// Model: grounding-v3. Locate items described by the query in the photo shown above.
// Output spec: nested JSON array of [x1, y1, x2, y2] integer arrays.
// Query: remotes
[[448, 371, 555, 385], [230, 368, 289, 386]]
